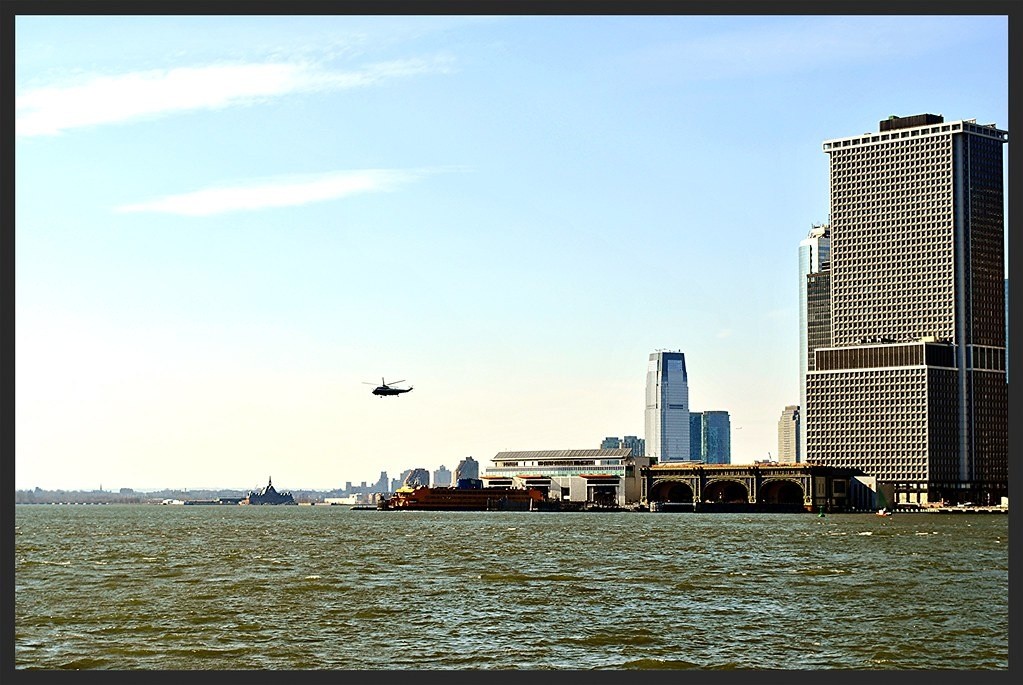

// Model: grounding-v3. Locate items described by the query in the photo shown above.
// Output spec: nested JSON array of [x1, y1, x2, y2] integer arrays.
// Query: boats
[[376, 480, 530, 512], [876, 507, 892, 517]]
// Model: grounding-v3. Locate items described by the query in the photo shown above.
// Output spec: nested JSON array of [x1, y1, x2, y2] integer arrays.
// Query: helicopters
[[361, 377, 415, 398]]
